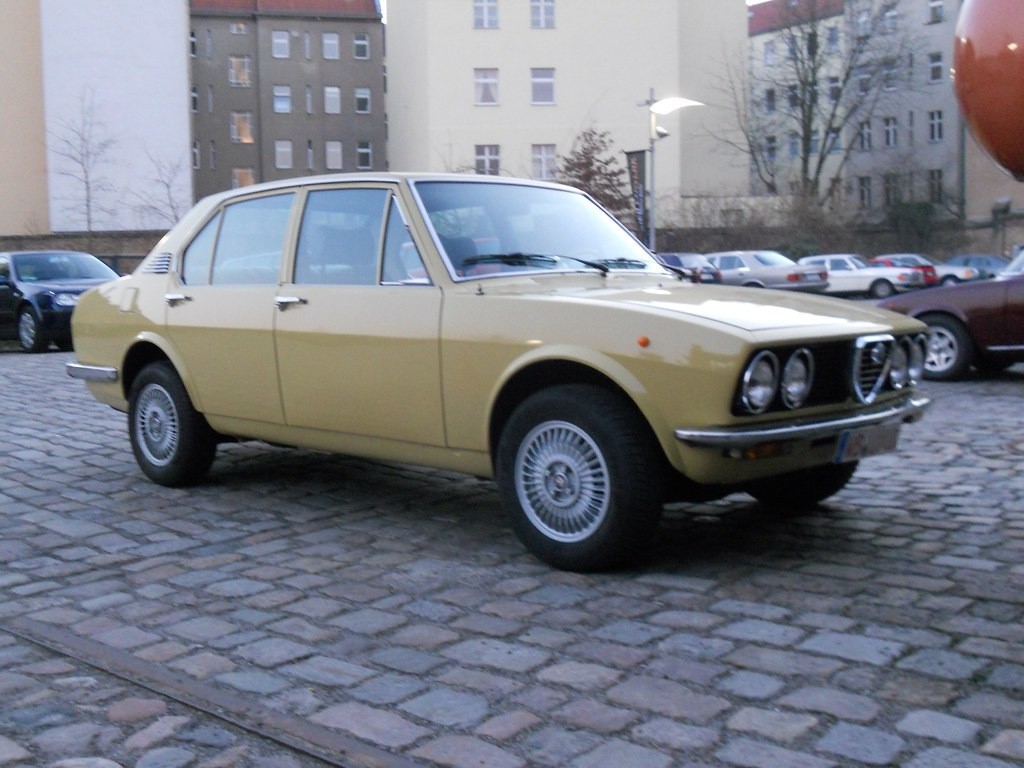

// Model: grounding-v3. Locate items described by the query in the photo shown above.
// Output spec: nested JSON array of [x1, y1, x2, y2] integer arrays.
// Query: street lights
[[648, 96, 704, 252]]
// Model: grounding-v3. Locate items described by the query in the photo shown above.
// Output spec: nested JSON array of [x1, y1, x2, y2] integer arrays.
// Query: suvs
[[654, 252, 722, 284]]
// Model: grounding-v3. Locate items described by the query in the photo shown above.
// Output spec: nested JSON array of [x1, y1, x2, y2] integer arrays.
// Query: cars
[[944, 255, 1011, 279], [870, 257, 939, 289], [879, 253, 981, 286], [874, 245, 1024, 382], [705, 250, 831, 293], [797, 253, 922, 299], [62, 171, 935, 570], [0, 250, 122, 353], [398, 236, 507, 277]]
